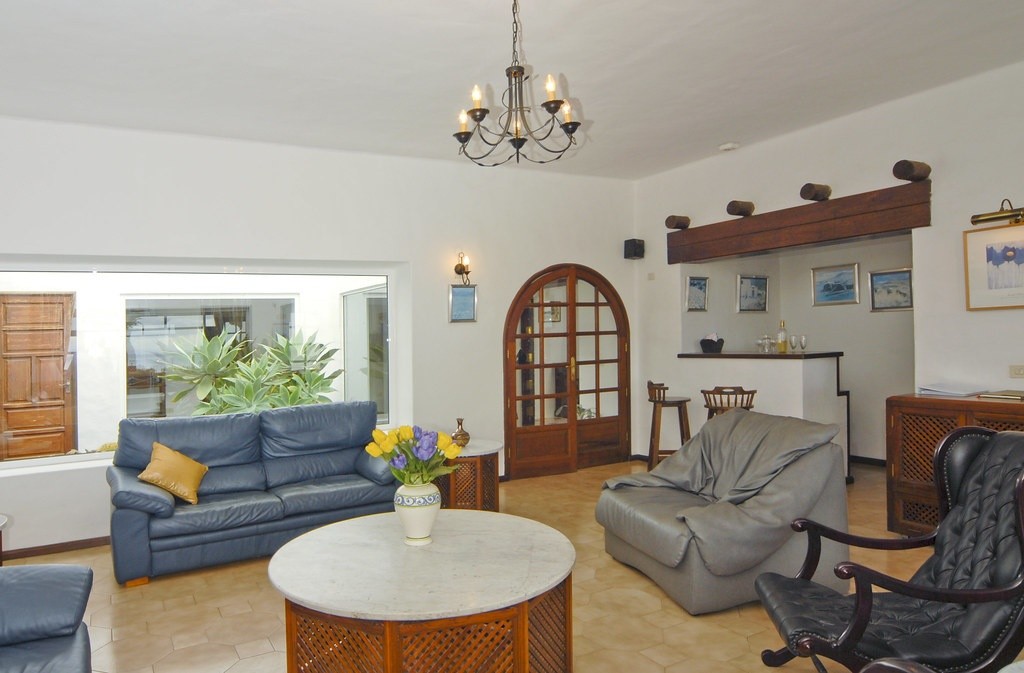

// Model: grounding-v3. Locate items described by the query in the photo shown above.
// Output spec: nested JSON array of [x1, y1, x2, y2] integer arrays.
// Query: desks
[[432, 439, 502, 512], [268, 509, 576, 673]]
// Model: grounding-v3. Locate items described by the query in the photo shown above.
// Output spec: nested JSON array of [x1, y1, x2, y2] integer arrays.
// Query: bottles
[[776, 320, 788, 353], [451, 417, 470, 446]]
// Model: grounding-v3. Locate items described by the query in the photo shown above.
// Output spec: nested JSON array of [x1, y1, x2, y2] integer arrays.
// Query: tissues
[[700, 333, 724, 353]]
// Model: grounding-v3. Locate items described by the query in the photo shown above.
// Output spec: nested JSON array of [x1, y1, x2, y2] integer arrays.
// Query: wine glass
[[800, 334, 807, 352], [789, 335, 798, 353]]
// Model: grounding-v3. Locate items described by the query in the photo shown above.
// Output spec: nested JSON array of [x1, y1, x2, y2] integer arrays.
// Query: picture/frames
[[684, 276, 709, 311], [963, 222, 1024, 311], [868, 267, 914, 312], [810, 263, 860, 307], [736, 273, 770, 312]]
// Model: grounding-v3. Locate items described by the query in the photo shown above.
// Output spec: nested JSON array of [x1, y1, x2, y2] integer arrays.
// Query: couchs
[[104, 400, 402, 588], [0, 564, 96, 673], [596, 406, 849, 615]]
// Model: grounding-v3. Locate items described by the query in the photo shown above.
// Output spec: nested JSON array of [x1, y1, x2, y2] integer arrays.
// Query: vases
[[451, 418, 470, 447], [394, 483, 441, 546]]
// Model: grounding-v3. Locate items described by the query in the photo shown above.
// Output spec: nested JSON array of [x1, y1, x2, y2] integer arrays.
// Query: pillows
[[136, 442, 209, 505]]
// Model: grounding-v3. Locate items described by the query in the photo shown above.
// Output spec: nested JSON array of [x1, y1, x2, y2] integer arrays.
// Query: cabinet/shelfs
[[885, 394, 1024, 535]]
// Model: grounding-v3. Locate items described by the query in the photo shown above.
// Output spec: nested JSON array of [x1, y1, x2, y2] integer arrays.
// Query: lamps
[[664, 160, 932, 228], [453, 0, 581, 166], [454, 252, 471, 287]]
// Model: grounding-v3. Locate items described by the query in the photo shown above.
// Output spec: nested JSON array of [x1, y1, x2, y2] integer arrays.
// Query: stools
[[701, 386, 757, 421], [646, 380, 691, 470]]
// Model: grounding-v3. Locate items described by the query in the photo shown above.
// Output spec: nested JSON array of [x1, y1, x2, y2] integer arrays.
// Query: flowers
[[365, 425, 461, 486]]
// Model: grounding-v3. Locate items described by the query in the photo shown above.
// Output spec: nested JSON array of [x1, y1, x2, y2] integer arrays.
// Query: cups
[[762, 337, 771, 353]]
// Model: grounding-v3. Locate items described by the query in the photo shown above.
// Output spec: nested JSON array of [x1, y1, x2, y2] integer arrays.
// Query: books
[[979, 390, 1024, 400]]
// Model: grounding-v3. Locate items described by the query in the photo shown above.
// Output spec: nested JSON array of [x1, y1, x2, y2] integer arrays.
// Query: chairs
[[754, 425, 1024, 673]]
